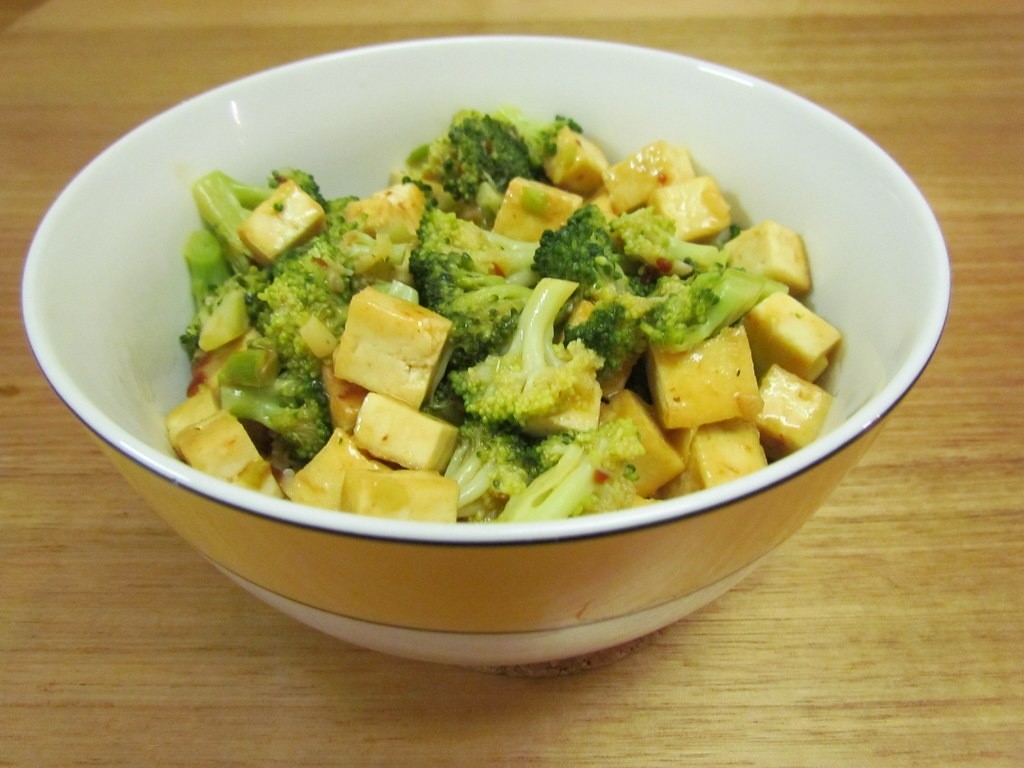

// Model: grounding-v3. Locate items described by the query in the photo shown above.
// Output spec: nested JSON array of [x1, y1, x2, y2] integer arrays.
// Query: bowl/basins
[[21, 35, 953, 667]]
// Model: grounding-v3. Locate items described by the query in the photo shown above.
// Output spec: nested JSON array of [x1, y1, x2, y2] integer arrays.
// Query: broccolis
[[181, 105, 789, 525]]
